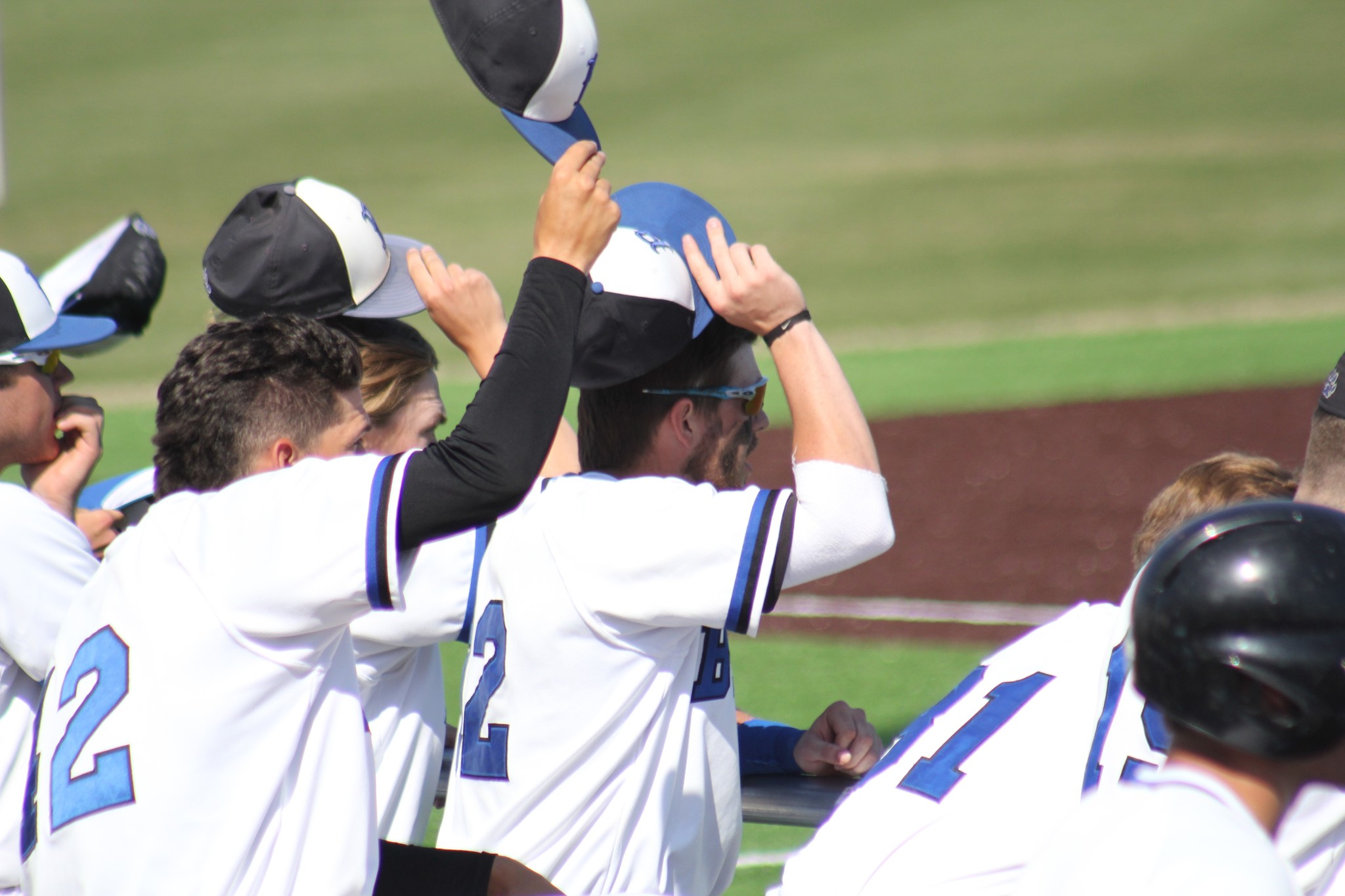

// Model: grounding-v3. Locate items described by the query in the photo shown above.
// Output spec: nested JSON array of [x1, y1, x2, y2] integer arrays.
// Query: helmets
[[1128, 495, 1338, 762]]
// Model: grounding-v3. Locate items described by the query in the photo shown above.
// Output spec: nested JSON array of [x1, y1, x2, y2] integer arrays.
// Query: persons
[[0, 136, 1345, 896]]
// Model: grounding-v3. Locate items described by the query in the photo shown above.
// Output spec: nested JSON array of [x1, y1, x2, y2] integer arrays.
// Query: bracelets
[[763, 309, 813, 347]]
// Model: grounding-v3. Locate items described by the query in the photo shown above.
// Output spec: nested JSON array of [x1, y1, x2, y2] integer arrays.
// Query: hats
[[571, 180, 748, 388], [0, 213, 170, 363], [199, 180, 449, 319], [421, 5, 603, 171]]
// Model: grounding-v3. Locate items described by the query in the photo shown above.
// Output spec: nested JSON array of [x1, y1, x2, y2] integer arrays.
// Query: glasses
[[639, 379, 769, 414], [3, 349, 59, 374]]
